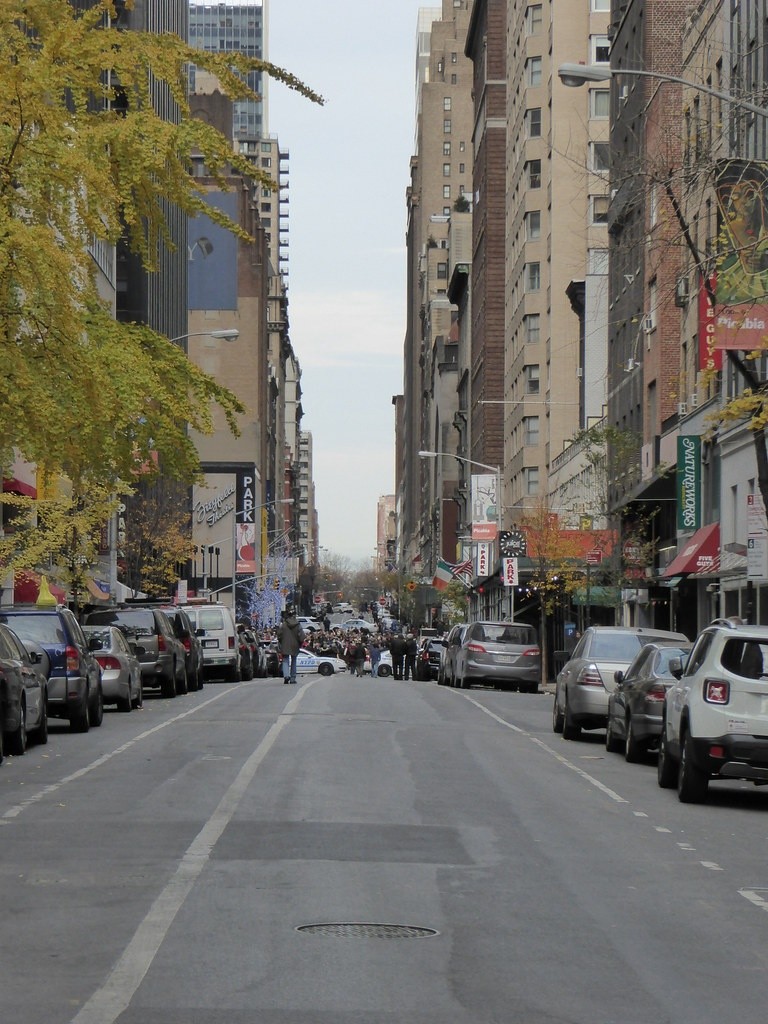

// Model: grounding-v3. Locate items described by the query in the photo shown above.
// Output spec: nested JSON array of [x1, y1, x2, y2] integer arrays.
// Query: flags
[[441, 557, 473, 577], [386, 561, 398, 576], [431, 559, 453, 590]]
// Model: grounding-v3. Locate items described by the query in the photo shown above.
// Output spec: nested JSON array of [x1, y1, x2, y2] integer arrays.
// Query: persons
[[311, 605, 334, 622], [359, 599, 420, 639], [422, 617, 448, 638], [323, 617, 330, 631], [275, 610, 306, 685], [405, 633, 418, 681], [301, 627, 392, 678], [390, 633, 406, 680]]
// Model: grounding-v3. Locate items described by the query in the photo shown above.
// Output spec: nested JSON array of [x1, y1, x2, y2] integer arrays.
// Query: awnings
[[13, 563, 66, 605], [572, 582, 622, 607], [661, 520, 722, 578]]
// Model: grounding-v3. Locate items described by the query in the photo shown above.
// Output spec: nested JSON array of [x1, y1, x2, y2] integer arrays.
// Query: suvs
[[118, 596, 206, 691], [657, 615, 768, 804], [0, 602, 106, 734], [81, 604, 191, 699], [172, 600, 242, 684]]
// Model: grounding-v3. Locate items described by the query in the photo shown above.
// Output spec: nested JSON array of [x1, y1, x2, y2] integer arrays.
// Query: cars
[[236, 598, 543, 695], [605, 641, 697, 763], [79, 624, 146, 713], [0, 622, 51, 756], [552, 624, 692, 740]]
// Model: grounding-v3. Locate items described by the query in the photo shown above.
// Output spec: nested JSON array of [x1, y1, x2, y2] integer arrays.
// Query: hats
[[407, 633, 414, 639], [288, 610, 295, 616]]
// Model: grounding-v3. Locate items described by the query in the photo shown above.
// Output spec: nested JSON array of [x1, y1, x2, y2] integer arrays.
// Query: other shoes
[[291, 677, 297, 684], [284, 677, 290, 684]]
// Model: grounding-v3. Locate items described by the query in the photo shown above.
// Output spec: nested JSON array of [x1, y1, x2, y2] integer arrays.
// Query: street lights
[[418, 449, 502, 532], [104, 329, 240, 610], [231, 498, 296, 609]]
